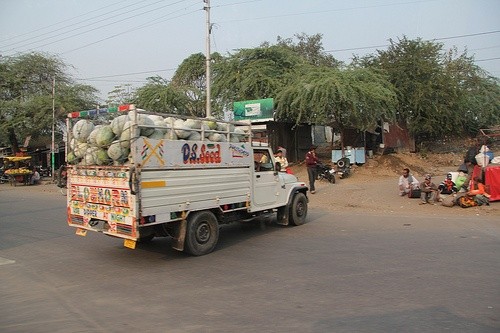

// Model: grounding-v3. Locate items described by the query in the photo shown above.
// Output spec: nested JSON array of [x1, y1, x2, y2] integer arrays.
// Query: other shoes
[[421, 201, 426, 205], [311, 190, 315, 193]]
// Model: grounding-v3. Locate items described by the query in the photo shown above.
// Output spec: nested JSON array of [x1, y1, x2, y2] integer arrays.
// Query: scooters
[[314, 159, 336, 184], [336, 155, 352, 180]]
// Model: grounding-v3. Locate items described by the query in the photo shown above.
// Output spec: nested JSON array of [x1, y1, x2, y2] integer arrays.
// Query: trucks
[[64, 103, 309, 257]]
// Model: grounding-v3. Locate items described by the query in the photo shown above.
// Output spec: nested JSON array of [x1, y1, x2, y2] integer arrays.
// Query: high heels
[[435, 198, 441, 202]]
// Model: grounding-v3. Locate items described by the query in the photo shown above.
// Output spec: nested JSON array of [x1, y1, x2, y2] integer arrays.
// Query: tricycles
[[9, 172, 30, 187]]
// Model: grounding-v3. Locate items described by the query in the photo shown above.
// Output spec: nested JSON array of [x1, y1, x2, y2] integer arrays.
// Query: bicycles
[[60, 180, 67, 195]]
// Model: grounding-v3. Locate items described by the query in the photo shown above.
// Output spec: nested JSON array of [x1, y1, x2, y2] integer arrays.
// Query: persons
[[454, 170, 468, 191], [29, 171, 40, 185], [399, 169, 417, 196], [275, 150, 289, 172], [259, 153, 268, 164], [466, 177, 491, 206], [57, 163, 66, 187], [437, 174, 458, 194], [276, 144, 288, 157], [419, 175, 438, 205], [305, 145, 320, 194]]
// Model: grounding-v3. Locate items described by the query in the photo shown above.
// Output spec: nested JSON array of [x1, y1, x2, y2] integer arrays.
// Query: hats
[[447, 173, 452, 180]]
